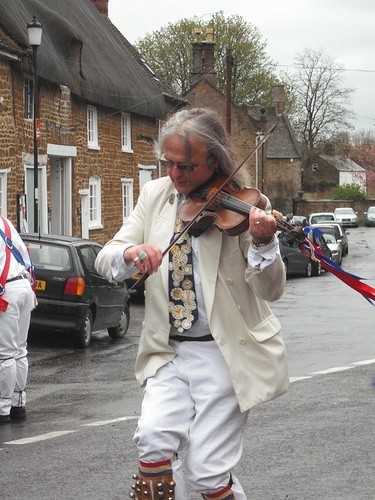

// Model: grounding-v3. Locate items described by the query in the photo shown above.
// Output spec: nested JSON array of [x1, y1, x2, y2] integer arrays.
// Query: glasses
[[159, 153, 213, 172]]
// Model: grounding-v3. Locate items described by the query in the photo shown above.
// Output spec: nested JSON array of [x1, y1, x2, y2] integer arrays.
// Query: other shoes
[[0, 406, 27, 423]]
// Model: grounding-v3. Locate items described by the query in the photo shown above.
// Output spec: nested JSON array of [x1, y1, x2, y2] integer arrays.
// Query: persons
[[0, 214, 38, 424], [94, 107, 289, 500]]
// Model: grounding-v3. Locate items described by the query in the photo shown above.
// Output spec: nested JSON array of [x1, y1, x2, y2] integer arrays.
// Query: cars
[[276, 212, 351, 277], [333, 207, 359, 227], [363, 206, 375, 227], [15, 232, 131, 349]]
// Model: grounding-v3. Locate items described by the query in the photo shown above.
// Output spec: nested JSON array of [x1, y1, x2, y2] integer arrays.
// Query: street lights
[[254, 127, 267, 195], [26, 14, 43, 231]]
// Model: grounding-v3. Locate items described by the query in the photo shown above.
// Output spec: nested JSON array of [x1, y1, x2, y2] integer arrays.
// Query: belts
[[6, 275, 27, 283]]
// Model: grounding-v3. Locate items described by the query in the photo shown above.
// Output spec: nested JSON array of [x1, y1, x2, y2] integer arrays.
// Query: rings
[[133, 251, 147, 262], [255, 221, 261, 224]]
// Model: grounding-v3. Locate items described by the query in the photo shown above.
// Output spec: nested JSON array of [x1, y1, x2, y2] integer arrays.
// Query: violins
[[178, 172, 310, 250]]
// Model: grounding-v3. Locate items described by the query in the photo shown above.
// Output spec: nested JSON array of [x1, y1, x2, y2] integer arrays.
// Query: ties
[[169, 196, 198, 333]]
